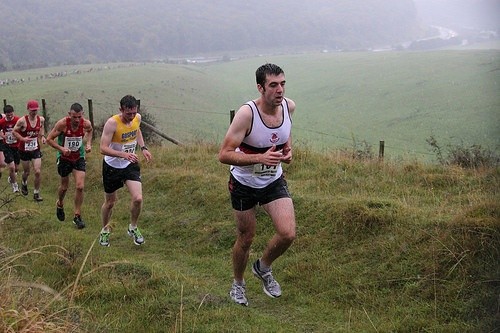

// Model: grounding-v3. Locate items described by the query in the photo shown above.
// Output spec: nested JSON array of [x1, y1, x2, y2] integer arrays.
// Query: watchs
[[141, 146, 148, 151]]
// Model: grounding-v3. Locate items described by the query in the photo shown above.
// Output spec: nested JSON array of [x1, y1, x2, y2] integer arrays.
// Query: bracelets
[[41, 134, 45, 137]]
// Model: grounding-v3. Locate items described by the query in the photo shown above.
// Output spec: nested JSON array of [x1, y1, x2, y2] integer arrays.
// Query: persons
[[0, 105, 22, 193], [12, 100, 46, 202], [46, 103, 93, 229], [0, 114, 7, 180], [219, 63, 296, 307], [99, 94, 153, 247]]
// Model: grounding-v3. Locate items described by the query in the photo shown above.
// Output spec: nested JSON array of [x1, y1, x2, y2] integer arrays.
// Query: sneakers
[[56, 199, 65, 221], [230, 283, 248, 306], [127, 223, 145, 245], [21, 180, 28, 195], [33, 193, 43, 201], [72, 216, 85, 226], [99, 228, 111, 247], [252, 257, 282, 297], [8, 170, 20, 183], [12, 182, 19, 192]]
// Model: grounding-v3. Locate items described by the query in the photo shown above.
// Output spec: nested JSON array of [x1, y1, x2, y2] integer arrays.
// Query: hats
[[27, 100, 39, 110]]
